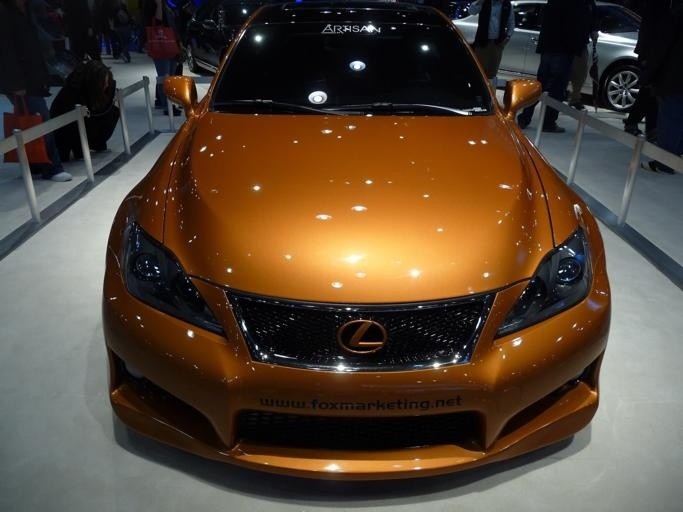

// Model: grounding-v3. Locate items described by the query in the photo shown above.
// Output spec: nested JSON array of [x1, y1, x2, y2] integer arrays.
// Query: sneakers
[[624, 126, 641, 135], [154, 100, 175, 109], [49, 171, 73, 182], [640, 160, 672, 175], [164, 107, 180, 116]]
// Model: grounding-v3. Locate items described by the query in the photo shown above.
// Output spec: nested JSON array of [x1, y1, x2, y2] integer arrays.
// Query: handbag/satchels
[[145, 17, 181, 59], [3, 94, 48, 164]]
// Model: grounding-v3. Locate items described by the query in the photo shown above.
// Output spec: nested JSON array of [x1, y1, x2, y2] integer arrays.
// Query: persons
[[622, 1, 657, 144], [516, 0, 600, 132], [638, 0, 683, 175], [567, 46, 589, 112], [466, 1, 515, 79], [0, 0, 194, 183]]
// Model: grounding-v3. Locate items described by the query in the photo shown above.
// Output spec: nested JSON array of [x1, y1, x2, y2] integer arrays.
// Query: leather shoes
[[518, 113, 526, 129], [543, 122, 564, 132]]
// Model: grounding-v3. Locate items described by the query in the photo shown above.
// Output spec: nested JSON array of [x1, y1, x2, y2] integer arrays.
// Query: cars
[[445, 1, 652, 113], [179, 0, 257, 77], [101, 2, 614, 485]]
